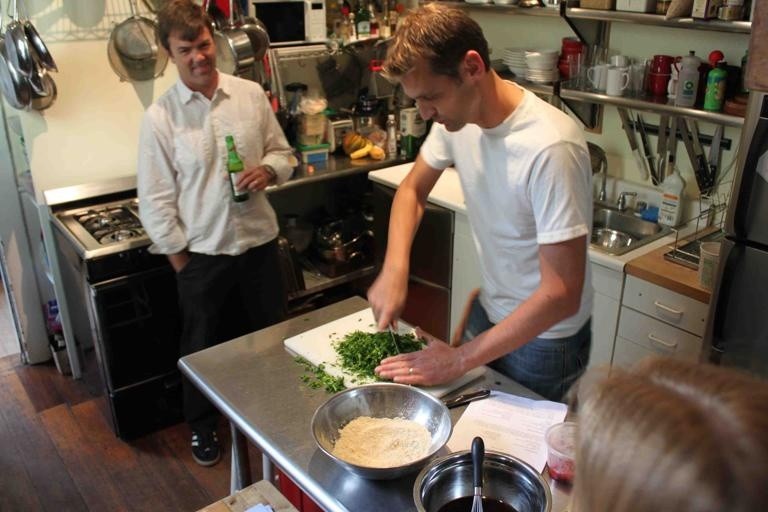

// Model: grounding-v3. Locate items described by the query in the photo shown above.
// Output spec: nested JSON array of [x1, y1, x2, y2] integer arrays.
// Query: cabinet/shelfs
[[448, 212, 625, 383], [419, 0, 753, 134], [613, 276, 709, 371]]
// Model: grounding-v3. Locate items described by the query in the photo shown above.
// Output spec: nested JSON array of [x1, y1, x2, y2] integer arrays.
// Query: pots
[[310, 212, 367, 266], [208, 0, 270, 74], [0, 0, 58, 114]]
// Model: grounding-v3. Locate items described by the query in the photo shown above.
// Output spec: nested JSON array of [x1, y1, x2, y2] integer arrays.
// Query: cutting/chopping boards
[[281, 307, 488, 400]]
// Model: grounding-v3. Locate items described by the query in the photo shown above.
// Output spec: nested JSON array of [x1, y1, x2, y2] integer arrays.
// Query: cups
[[696, 242, 721, 289], [560, 37, 682, 101], [285, 80, 307, 111], [542, 421, 577, 485]]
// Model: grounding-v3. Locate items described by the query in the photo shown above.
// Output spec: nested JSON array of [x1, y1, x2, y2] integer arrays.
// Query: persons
[[367, 3, 591, 401], [567, 357, 767, 512], [136, 1, 295, 466]]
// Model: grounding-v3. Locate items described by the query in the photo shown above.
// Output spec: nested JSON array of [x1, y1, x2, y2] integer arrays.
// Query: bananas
[[349, 137, 373, 159]]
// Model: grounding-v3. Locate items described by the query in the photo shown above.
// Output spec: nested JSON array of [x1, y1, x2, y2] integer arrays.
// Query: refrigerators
[[703, 88, 767, 383]]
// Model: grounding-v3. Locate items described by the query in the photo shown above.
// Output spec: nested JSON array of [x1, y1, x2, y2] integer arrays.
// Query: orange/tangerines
[[369, 145, 385, 160]]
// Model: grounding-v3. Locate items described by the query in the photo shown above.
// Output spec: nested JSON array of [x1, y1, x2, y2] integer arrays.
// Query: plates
[[500, 45, 562, 85]]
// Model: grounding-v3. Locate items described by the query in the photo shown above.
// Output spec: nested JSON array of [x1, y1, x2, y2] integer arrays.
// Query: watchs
[[265, 165, 276, 178]]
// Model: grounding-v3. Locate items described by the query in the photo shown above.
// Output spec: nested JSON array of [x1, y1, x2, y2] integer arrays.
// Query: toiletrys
[[658, 168, 687, 228]]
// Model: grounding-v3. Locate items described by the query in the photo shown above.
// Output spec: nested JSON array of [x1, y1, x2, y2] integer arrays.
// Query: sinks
[[590, 209, 670, 257]]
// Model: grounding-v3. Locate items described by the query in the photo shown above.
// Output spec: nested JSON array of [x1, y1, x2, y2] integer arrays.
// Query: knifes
[[383, 318, 403, 355], [614, 106, 709, 193], [704, 124, 724, 194]]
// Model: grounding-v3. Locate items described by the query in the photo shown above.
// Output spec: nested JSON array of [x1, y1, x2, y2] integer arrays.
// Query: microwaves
[[246, 0, 327, 46]]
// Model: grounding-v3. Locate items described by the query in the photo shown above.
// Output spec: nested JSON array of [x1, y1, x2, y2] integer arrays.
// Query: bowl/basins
[[413, 448, 551, 512], [464, 0, 562, 7], [307, 381, 453, 481]]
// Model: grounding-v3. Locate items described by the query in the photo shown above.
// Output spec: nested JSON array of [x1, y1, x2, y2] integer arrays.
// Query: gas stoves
[[46, 189, 173, 283]]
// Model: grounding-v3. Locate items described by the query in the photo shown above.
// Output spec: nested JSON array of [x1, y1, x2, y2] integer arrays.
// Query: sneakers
[[190, 429, 221, 465]]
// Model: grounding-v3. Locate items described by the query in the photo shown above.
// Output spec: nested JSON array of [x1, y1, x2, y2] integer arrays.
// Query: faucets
[[589, 144, 608, 200]]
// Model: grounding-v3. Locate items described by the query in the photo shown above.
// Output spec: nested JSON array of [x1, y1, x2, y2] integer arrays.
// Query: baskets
[[666, 181, 730, 264]]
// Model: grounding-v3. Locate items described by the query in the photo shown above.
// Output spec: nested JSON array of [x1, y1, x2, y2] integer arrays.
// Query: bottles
[[223, 134, 250, 203], [262, 81, 279, 117], [352, 93, 381, 132], [384, 110, 398, 155], [324, 0, 406, 41], [736, 48, 750, 94], [702, 59, 727, 111]]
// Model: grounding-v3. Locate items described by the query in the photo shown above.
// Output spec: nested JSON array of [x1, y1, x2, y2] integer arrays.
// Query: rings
[[408, 366, 412, 373]]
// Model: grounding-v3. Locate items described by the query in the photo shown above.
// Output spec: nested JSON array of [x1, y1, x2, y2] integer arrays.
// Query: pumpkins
[[342, 132, 365, 154]]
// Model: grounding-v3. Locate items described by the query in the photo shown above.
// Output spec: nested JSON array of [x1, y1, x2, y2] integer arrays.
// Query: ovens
[[52, 246, 187, 444]]
